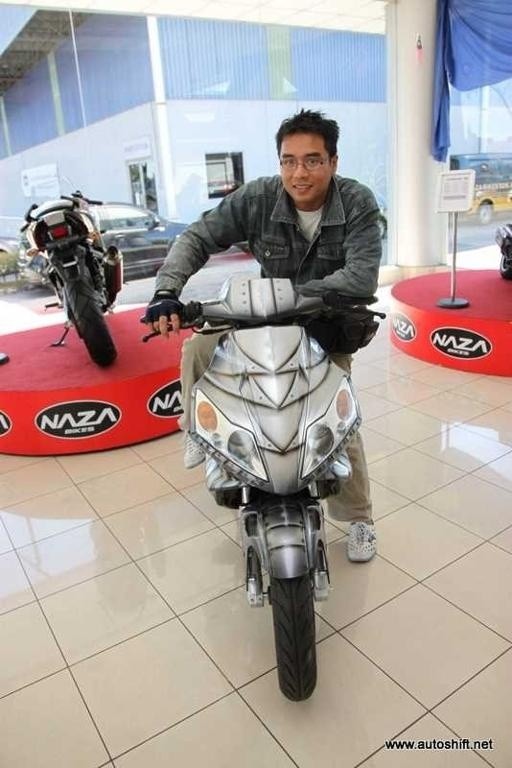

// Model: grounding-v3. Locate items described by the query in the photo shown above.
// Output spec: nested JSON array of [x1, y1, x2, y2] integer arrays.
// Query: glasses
[[280, 156, 327, 169]]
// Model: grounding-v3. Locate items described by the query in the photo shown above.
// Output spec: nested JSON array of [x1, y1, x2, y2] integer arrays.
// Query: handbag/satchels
[[307, 306, 379, 354]]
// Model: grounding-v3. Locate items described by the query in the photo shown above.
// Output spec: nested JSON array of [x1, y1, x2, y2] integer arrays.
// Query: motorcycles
[[493, 223, 512, 278], [139, 273, 390, 702], [19, 187, 127, 367]]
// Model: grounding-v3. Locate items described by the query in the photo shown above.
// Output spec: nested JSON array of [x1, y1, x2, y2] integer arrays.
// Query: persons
[[140, 108, 385, 563]]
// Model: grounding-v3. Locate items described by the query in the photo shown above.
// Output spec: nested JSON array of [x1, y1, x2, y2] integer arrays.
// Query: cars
[[448, 152, 511, 227], [22, 202, 192, 293]]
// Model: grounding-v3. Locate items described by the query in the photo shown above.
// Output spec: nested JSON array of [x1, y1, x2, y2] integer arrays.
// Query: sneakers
[[185, 433, 206, 469], [347, 521, 376, 561]]
